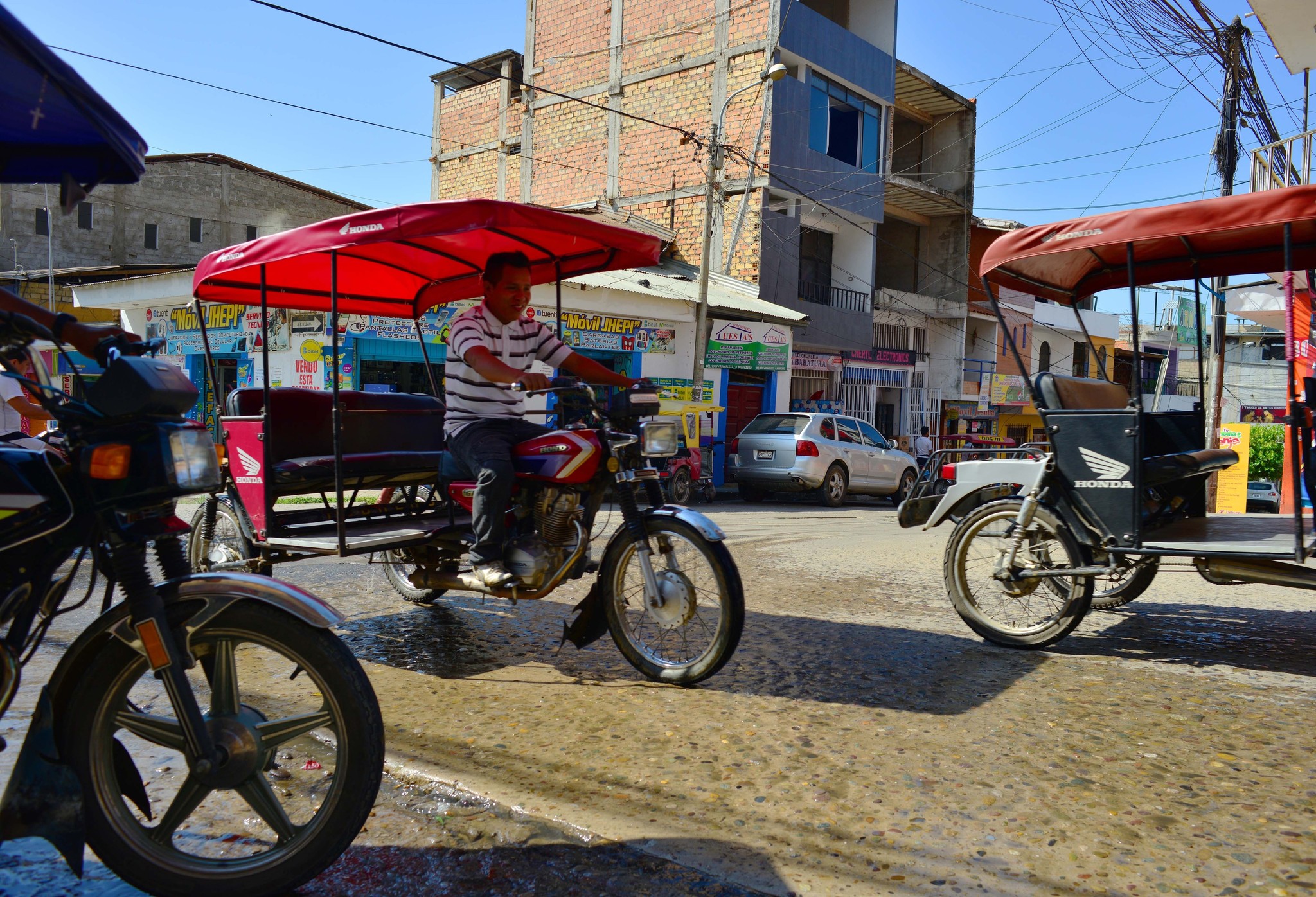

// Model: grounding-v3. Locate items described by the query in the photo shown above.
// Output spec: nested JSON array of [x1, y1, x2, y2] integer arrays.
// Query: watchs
[[53, 312, 77, 344]]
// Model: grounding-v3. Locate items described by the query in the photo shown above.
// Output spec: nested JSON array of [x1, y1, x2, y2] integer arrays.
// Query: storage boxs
[[889, 435, 914, 457]]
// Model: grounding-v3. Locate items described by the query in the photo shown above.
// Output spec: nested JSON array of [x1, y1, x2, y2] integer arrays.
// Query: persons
[[0, 287, 143, 360], [915, 426, 933, 470], [0, 346, 63, 458], [1243, 409, 1274, 423], [441, 249, 653, 589], [961, 442, 974, 462]]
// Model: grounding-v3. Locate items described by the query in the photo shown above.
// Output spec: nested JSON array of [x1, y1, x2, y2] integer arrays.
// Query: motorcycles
[[937, 432, 1017, 520], [0, 288, 386, 897], [635, 399, 726, 506], [898, 181, 1316, 648], [189, 197, 745, 686]]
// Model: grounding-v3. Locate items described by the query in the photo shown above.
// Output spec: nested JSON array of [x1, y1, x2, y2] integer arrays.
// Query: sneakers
[[472, 560, 514, 587]]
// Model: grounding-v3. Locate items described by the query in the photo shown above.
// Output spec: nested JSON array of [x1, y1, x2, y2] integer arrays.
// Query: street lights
[[693, 63, 789, 407]]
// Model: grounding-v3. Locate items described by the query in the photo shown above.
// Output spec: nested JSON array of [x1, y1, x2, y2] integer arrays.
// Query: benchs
[[225, 387, 447, 529], [1028, 372, 1239, 545]]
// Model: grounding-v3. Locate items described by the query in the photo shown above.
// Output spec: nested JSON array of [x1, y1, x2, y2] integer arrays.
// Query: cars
[[728, 411, 920, 507]]
